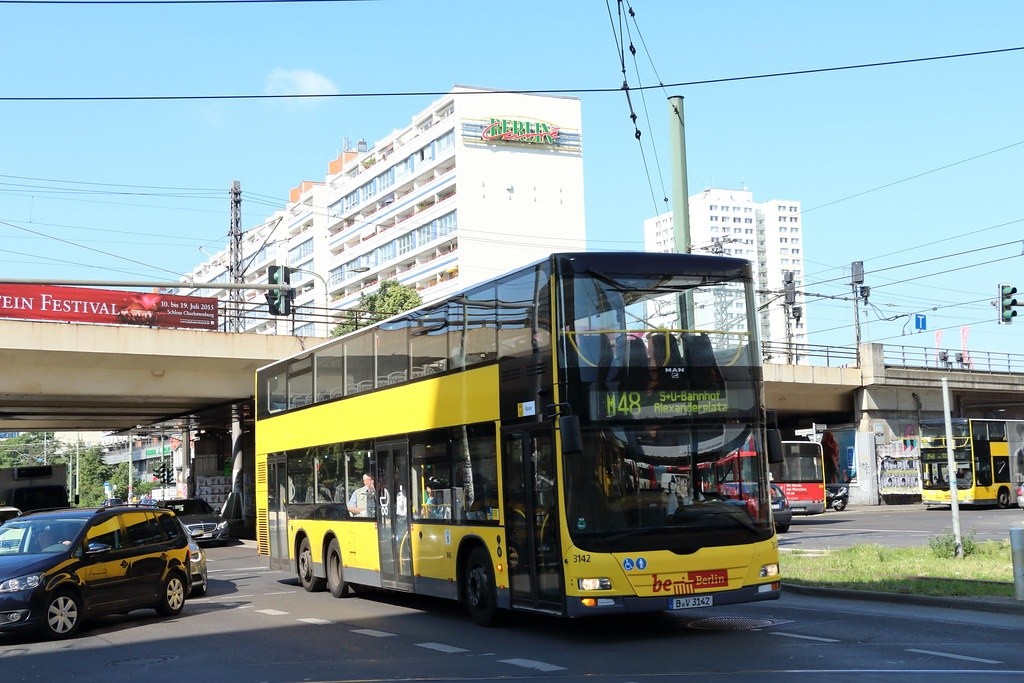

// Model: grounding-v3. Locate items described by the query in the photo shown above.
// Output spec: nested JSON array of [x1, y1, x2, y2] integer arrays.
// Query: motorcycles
[[825, 487, 849, 513]]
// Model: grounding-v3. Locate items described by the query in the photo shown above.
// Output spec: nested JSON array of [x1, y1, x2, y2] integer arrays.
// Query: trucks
[[0, 463, 79, 513]]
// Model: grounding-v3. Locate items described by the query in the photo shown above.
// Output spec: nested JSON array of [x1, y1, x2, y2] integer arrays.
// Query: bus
[[768, 441, 826, 516], [919, 418, 1024, 509], [253, 251, 784, 627], [622, 452, 757, 496]]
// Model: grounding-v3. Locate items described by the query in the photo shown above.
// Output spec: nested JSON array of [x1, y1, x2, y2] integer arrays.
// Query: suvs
[[0, 504, 193, 641]]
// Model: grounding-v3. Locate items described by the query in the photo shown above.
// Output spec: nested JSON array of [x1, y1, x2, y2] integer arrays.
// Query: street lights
[[288, 267, 371, 337]]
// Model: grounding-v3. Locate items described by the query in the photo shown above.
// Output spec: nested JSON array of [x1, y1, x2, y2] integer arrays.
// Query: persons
[[62, 525, 95, 548], [903, 424, 915, 451], [820, 429, 840, 509], [1013, 423, 1024, 482], [36, 528, 58, 553], [347, 472, 376, 518], [666, 473, 705, 518]]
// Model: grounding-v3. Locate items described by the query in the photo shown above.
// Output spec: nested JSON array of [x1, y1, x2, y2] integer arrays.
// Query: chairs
[[615, 334, 647, 366], [646, 332, 681, 366], [305, 482, 357, 502], [579, 329, 613, 367], [681, 332, 717, 365], [292, 362, 445, 407]]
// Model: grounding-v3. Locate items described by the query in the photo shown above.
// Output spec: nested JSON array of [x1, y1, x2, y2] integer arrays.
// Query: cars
[[723, 482, 792, 533], [177, 517, 208, 597], [138, 499, 158, 508], [0, 506, 32, 554], [1017, 483, 1024, 508], [152, 500, 230, 544], [99, 498, 124, 508]]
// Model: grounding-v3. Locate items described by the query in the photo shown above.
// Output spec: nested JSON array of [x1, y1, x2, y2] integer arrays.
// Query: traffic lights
[[998, 284, 1018, 325], [159, 463, 166, 484], [153, 468, 160, 479], [166, 468, 174, 484]]
